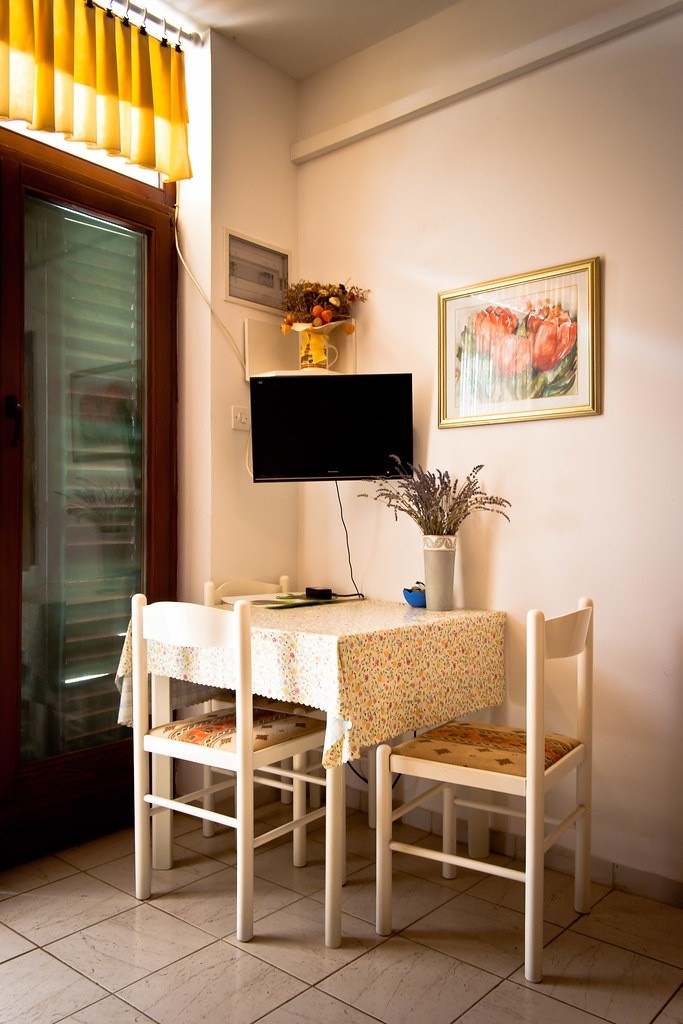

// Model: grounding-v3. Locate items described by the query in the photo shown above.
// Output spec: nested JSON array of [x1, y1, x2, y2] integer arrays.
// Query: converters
[[307, 587, 332, 600]]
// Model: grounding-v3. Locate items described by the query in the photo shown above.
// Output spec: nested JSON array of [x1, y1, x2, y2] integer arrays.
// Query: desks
[[113, 596, 505, 950]]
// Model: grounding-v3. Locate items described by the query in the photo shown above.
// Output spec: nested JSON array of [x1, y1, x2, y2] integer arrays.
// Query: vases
[[424, 533, 454, 611], [291, 318, 348, 370]]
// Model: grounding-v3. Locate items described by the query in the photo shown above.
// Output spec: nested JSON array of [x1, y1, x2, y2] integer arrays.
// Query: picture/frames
[[437, 256, 601, 425]]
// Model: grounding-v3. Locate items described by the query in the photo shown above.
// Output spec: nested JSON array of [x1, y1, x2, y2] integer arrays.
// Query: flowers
[[279, 276, 370, 325], [356, 451, 512, 534]]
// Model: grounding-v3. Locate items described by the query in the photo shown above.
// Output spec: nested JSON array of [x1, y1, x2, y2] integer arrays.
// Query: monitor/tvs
[[249, 373, 414, 483]]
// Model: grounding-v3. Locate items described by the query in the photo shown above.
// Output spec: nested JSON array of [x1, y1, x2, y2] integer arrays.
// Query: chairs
[[377, 595, 595, 984], [202, 574, 324, 838], [131, 591, 326, 941]]
